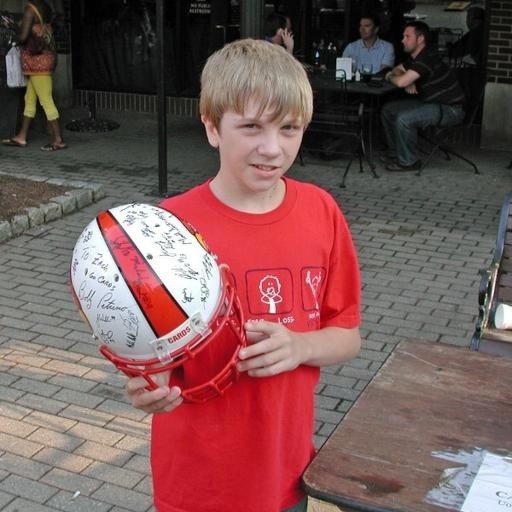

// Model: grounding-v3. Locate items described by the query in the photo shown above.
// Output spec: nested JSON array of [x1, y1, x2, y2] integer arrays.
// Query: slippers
[[3, 138, 28, 148], [41, 141, 67, 151]]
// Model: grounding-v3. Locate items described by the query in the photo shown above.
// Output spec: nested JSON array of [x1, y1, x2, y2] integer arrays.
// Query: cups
[[348, 55, 358, 72], [362, 63, 374, 81], [494, 303, 512, 330]]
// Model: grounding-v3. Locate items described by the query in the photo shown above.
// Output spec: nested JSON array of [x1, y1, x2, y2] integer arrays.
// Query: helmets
[[70, 205, 248, 403]]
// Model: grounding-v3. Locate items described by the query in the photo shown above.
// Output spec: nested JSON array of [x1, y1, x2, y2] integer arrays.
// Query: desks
[[300, 337, 511, 512]]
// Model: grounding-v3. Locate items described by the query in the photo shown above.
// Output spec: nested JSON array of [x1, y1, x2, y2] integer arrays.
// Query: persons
[[448, 7, 484, 124], [380, 21, 466, 172], [0, 0, 67, 152], [264, 12, 294, 55], [125, 38, 362, 512], [319, 14, 395, 160]]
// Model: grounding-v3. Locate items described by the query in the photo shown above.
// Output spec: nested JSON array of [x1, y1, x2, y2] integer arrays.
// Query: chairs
[[299, 103, 379, 188], [415, 69, 489, 176], [470, 192, 511, 352]]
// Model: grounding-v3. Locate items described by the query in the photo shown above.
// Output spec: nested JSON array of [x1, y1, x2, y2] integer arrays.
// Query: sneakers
[[379, 152, 431, 170]]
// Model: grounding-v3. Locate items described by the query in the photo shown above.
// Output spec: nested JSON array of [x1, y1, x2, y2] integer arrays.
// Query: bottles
[[312, 40, 338, 69], [314, 58, 321, 71], [355, 69, 361, 81]]
